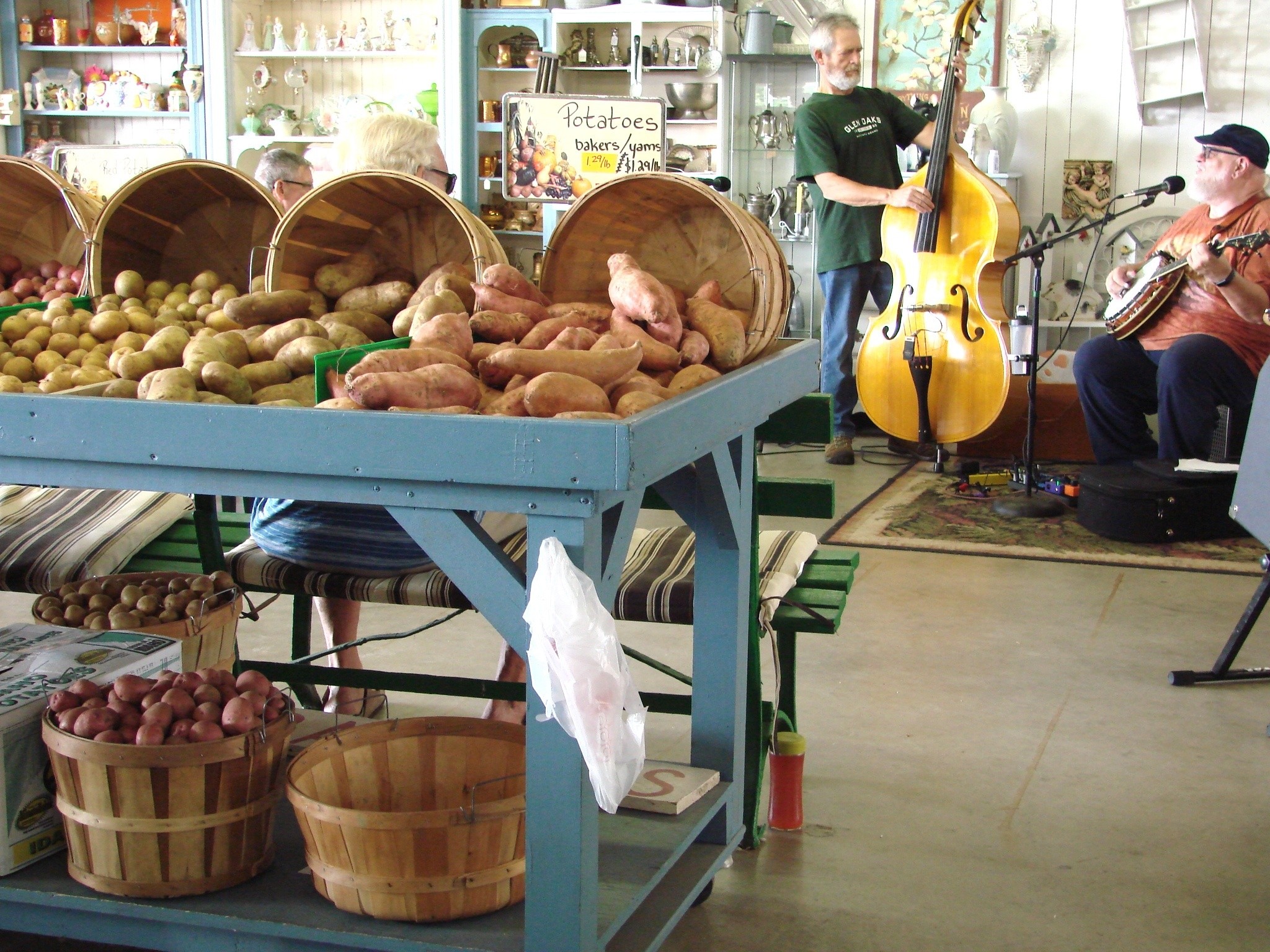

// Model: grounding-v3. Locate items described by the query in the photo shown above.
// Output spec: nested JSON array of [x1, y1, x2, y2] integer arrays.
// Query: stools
[[958, 376, 1096, 462]]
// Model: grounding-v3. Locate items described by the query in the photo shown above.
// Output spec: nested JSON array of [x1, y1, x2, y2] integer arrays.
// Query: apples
[[507, 148, 576, 196]]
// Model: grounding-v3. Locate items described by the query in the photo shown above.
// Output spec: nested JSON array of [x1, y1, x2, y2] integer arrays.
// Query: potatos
[[38, 571, 233, 630], [0, 253, 476, 409], [51, 670, 285, 745], [312, 253, 754, 417]]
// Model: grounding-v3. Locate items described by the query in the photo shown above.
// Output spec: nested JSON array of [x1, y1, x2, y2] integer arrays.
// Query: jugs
[[747, 97, 807, 149], [736, 174, 813, 239], [733, 6, 795, 54]]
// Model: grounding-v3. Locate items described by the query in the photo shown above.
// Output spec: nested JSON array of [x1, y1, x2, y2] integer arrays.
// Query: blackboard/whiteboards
[[504, 90, 664, 205]]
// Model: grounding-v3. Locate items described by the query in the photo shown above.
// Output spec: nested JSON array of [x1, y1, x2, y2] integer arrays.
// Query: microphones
[[692, 177, 731, 192], [1116, 176, 1186, 199]]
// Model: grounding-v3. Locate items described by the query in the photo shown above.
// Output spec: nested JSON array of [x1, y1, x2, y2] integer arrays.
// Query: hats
[[1194, 123, 1269, 169]]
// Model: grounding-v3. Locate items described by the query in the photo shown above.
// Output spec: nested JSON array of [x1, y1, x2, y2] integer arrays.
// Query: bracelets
[[1213, 266, 1235, 287]]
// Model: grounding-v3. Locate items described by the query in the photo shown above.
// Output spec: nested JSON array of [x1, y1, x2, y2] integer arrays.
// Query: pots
[[487, 32, 539, 68]]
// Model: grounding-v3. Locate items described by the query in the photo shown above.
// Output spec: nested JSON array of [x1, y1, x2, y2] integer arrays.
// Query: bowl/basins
[[93, 22, 135, 45], [664, 83, 717, 119]]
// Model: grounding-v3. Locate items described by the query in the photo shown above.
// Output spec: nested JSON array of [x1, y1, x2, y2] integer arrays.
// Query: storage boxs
[[0, 624, 182, 881]]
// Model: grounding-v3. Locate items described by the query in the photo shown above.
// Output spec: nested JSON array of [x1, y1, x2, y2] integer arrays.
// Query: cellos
[[855, 0, 1026, 473]]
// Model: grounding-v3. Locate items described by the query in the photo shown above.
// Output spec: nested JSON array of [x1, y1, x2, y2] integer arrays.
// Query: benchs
[[126, 392, 860, 848]]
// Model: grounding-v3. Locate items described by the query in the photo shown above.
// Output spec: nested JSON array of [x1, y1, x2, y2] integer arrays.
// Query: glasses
[[282, 177, 320, 194], [428, 167, 461, 196], [1201, 145, 1243, 159]]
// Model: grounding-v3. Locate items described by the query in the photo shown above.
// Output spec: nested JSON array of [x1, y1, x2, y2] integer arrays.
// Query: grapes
[[546, 178, 571, 199]]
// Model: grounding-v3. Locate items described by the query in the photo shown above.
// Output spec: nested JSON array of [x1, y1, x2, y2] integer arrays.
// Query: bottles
[[766, 731, 805, 831], [168, 90, 189, 112], [559, 26, 624, 67], [527, 252, 542, 291], [17, 8, 55, 45], [963, 85, 1019, 173]]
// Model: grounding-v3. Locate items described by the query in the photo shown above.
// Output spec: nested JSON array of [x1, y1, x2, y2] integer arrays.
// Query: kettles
[[786, 263, 805, 331]]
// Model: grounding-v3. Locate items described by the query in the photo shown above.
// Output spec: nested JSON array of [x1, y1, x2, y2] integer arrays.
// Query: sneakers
[[886, 433, 950, 463], [824, 436, 856, 466]]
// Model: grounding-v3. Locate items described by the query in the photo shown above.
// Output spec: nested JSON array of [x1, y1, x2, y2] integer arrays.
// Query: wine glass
[[77, 28, 90, 45]]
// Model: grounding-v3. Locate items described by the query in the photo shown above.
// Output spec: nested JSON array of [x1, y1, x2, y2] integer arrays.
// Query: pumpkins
[[571, 175, 592, 198], [516, 165, 536, 185], [532, 149, 556, 173]]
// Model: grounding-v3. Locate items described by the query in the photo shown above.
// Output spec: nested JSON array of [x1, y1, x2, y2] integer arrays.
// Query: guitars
[[1102, 226, 1270, 343]]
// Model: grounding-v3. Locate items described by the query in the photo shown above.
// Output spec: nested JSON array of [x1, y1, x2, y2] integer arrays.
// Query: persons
[[252, 114, 524, 728], [794, 14, 966, 465], [255, 149, 313, 213], [1073, 124, 1270, 462], [236, 9, 438, 52]]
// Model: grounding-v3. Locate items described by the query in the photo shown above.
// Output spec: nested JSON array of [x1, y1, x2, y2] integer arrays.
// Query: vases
[[970, 86, 1018, 174]]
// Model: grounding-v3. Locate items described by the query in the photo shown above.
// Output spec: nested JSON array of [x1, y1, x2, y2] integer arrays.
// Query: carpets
[[817, 457, 1270, 578]]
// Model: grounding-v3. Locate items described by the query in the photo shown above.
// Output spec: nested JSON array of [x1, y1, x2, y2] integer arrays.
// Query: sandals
[[355, 688, 386, 719]]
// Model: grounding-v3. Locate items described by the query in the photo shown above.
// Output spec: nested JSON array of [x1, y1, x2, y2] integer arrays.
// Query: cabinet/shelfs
[[0, 339, 826, 952], [1123, 0, 1212, 126], [0, 0, 821, 338]]
